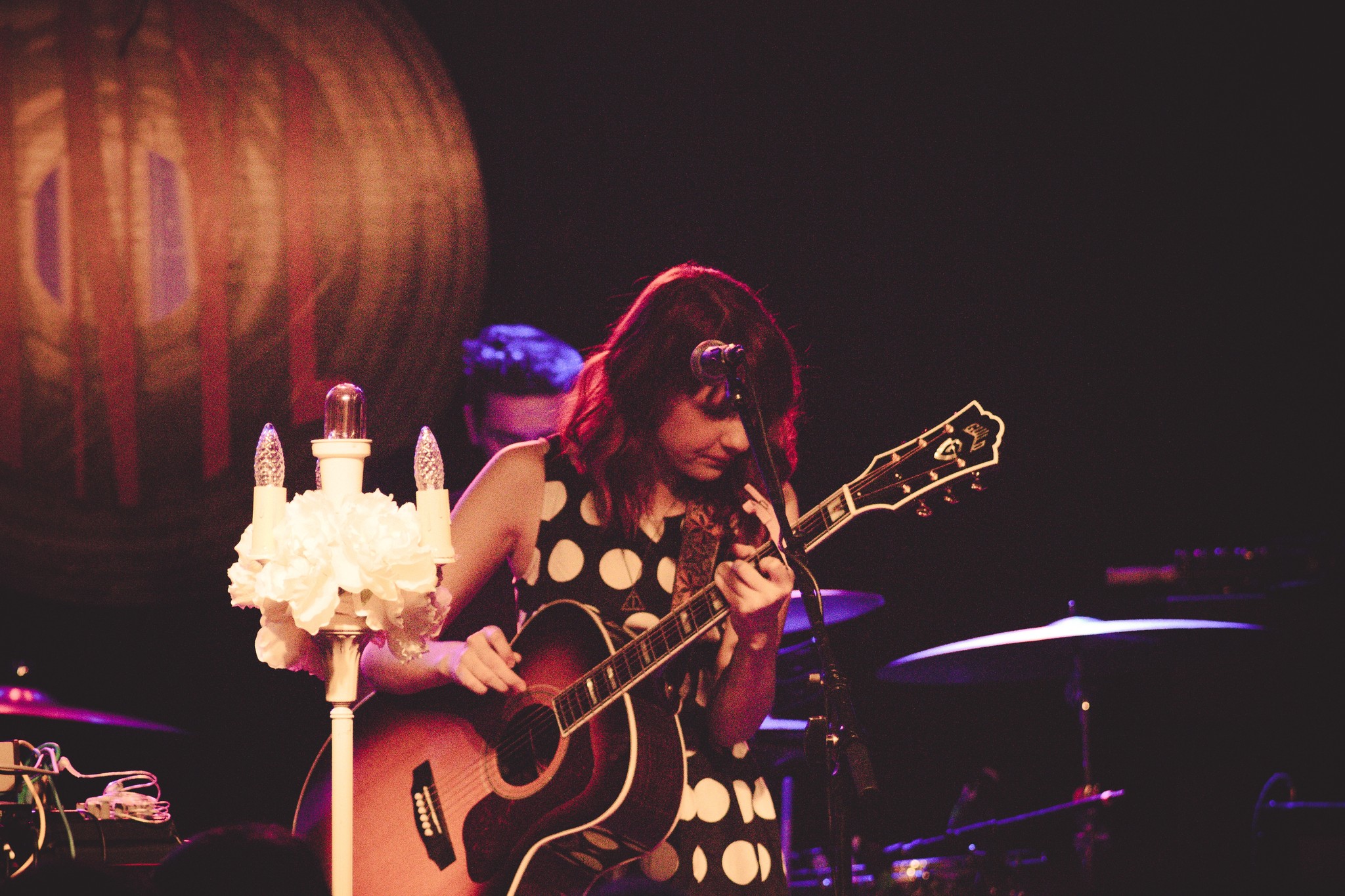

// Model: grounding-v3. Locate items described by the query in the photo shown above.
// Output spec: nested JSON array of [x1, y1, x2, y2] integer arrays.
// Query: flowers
[[228, 487, 437, 672]]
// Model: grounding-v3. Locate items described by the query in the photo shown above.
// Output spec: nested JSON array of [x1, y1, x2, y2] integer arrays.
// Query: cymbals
[[877, 614, 1266, 685], [758, 712, 806, 731], [0, 684, 178, 735], [783, 589, 885, 636]]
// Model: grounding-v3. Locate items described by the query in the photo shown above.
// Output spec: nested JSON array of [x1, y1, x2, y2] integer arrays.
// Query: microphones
[[691, 339, 745, 386]]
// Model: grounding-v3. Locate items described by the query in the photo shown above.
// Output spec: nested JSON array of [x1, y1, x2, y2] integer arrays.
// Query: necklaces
[[618, 483, 692, 612]]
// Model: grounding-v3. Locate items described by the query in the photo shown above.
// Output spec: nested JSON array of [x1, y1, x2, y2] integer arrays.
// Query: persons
[[143, 822, 330, 896], [359, 264, 804, 895], [406, 326, 587, 645]]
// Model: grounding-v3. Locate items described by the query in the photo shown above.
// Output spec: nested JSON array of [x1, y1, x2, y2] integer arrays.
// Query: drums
[[785, 856, 992, 895]]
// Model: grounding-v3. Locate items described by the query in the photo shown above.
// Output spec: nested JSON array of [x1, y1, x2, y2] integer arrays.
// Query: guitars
[[289, 399, 1006, 896]]
